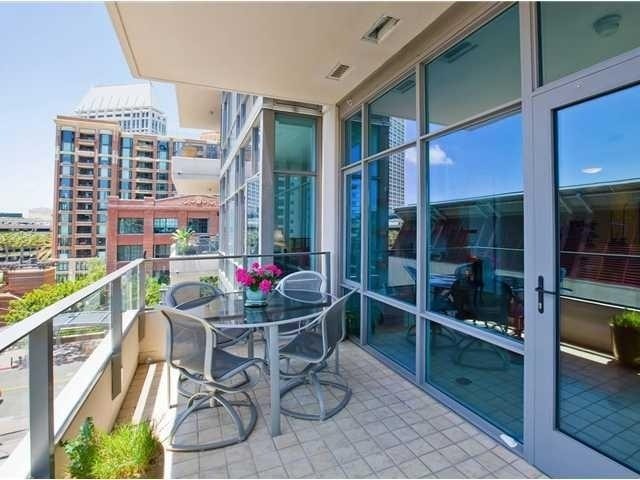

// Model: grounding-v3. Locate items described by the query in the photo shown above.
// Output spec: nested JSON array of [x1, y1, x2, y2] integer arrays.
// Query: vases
[[243, 286, 269, 309]]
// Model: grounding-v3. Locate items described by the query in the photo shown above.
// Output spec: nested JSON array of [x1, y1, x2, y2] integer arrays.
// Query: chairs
[[256, 268, 330, 381], [277, 286, 364, 424], [400, 255, 567, 375], [164, 279, 256, 400], [158, 306, 260, 453]]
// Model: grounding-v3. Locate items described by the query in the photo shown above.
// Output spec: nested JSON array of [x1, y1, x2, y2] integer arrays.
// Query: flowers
[[232, 259, 284, 296]]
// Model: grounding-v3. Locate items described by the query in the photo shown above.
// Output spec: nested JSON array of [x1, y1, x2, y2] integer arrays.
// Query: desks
[[173, 286, 344, 440]]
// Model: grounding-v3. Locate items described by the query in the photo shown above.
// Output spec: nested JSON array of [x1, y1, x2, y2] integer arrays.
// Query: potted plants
[[64, 415, 166, 479], [607, 308, 640, 369]]
